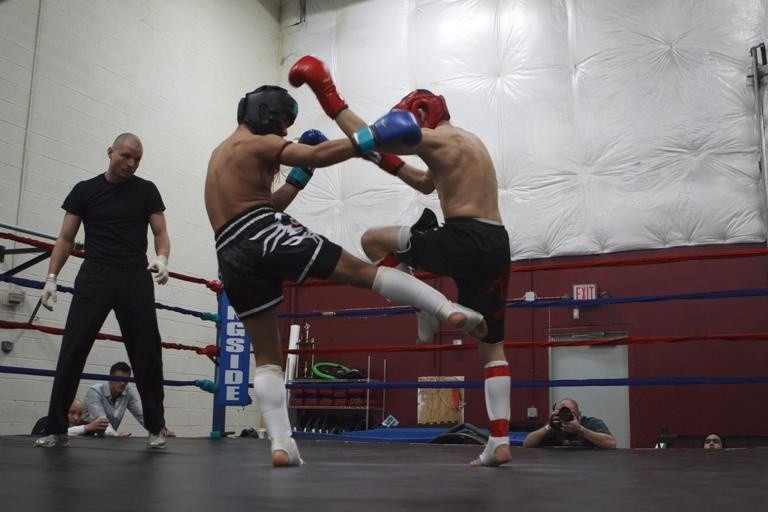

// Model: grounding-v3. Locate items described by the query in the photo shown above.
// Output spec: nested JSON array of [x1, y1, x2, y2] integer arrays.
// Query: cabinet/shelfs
[[287, 356, 386, 431]]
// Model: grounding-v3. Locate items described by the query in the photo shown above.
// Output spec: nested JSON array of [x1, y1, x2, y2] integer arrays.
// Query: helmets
[[392, 87, 452, 128], [235, 83, 299, 137]]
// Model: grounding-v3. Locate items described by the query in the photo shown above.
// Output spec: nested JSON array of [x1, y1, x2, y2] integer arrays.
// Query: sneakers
[[143, 428, 170, 450], [28, 428, 59, 450]]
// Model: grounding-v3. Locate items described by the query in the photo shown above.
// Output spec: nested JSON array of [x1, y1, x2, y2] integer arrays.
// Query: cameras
[[554, 406, 574, 429]]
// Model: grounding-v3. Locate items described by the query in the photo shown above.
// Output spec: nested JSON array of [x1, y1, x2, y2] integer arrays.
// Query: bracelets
[[47, 273, 57, 278], [545, 424, 552, 431]]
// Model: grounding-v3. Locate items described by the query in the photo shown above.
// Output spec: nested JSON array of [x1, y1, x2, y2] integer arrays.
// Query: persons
[[31, 131, 172, 449], [81, 361, 176, 437], [32, 396, 109, 436], [522, 397, 617, 449], [701, 431, 727, 450], [286, 54, 516, 470], [202, 84, 489, 469]]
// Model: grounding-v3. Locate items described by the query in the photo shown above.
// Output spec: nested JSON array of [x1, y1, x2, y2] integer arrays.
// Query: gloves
[[146, 255, 171, 286], [286, 128, 332, 191], [39, 277, 59, 312], [287, 53, 349, 120], [362, 148, 405, 177], [350, 107, 424, 153]]
